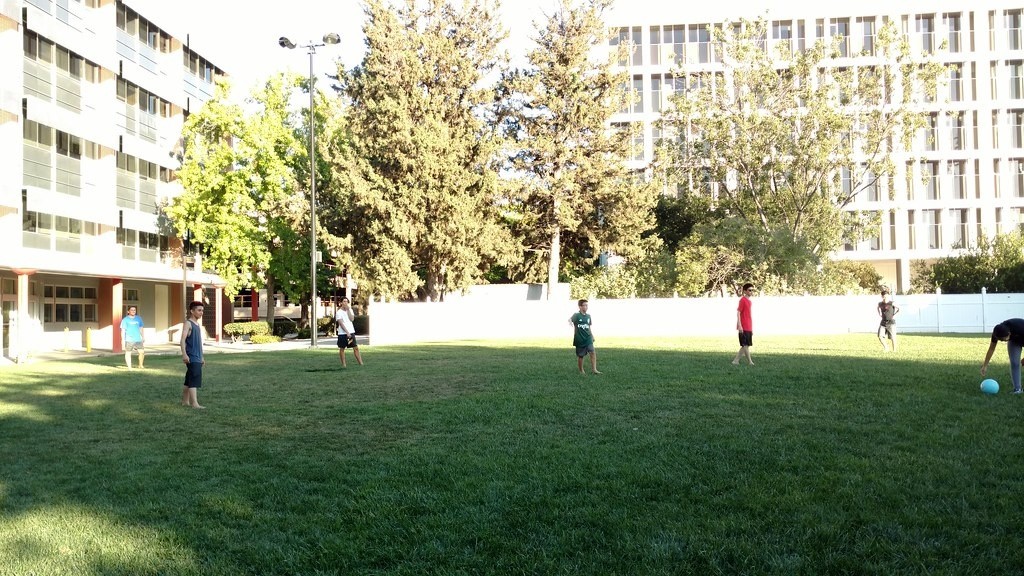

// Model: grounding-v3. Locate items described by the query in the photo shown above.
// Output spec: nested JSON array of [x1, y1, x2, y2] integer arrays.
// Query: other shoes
[[1010, 389, 1023, 394]]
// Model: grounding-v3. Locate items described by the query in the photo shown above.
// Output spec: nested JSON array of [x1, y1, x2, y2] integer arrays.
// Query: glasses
[[747, 289, 754, 292]]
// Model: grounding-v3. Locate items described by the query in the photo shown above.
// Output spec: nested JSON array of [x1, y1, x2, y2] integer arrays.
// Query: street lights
[[277, 32, 342, 348]]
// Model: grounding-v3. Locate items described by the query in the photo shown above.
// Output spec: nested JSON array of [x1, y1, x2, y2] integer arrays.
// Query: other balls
[[980, 378, 1000, 394]]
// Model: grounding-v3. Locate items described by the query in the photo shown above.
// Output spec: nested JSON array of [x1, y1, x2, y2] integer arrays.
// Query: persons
[[732, 283, 755, 366], [981, 318, 1024, 395], [120, 306, 146, 369], [878, 291, 900, 353], [180, 301, 206, 410], [336, 296, 363, 367], [568, 300, 602, 375]]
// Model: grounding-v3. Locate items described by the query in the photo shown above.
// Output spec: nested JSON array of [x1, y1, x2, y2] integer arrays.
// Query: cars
[[273, 316, 297, 338]]
